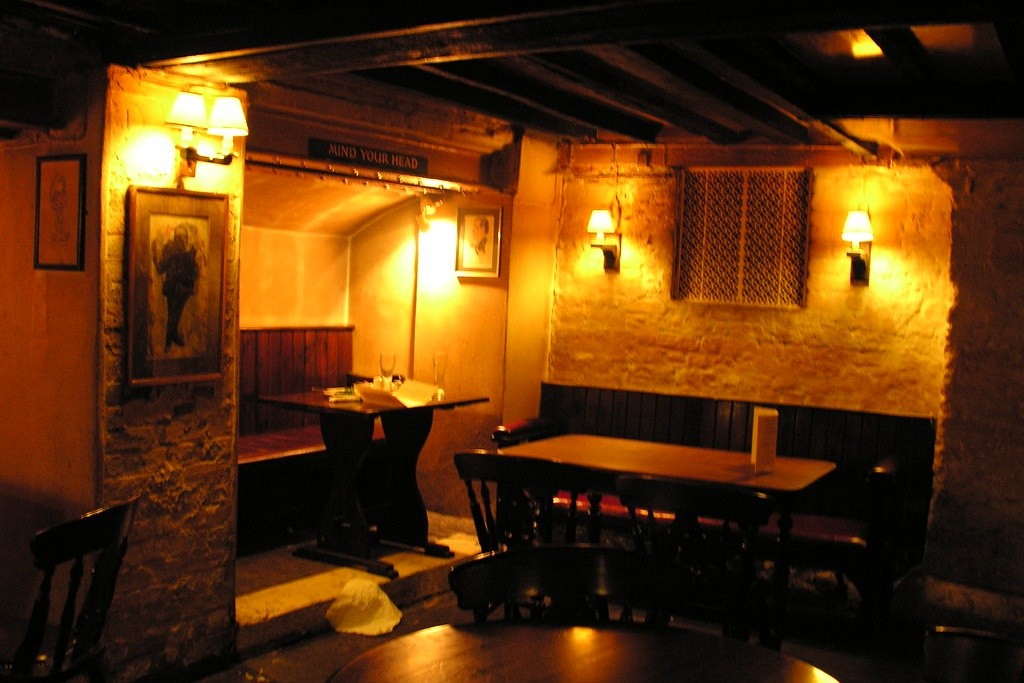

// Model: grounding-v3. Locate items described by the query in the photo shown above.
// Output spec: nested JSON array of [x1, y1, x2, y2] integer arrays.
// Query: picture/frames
[[33, 154, 87, 273], [127, 186, 229, 387], [454, 206, 504, 279]]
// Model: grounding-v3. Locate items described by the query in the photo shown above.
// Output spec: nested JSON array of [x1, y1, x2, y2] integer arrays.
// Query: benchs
[[456, 376, 937, 658], [235, 324, 387, 467]]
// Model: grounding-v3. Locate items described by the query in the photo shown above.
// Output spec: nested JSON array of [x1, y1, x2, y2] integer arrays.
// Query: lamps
[[163, 90, 248, 165], [843, 209, 874, 285], [587, 209, 622, 271]]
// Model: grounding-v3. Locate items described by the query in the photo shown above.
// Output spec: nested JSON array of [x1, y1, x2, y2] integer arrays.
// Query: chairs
[[0, 494, 143, 683], [444, 545, 674, 625]]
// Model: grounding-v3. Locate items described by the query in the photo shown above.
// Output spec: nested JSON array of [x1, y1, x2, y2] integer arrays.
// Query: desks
[[327, 620, 844, 683], [496, 430, 839, 652], [238, 381, 493, 579]]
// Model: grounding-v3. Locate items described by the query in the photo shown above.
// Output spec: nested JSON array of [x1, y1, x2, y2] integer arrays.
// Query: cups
[[380, 352, 396, 382], [433, 350, 447, 387]]
